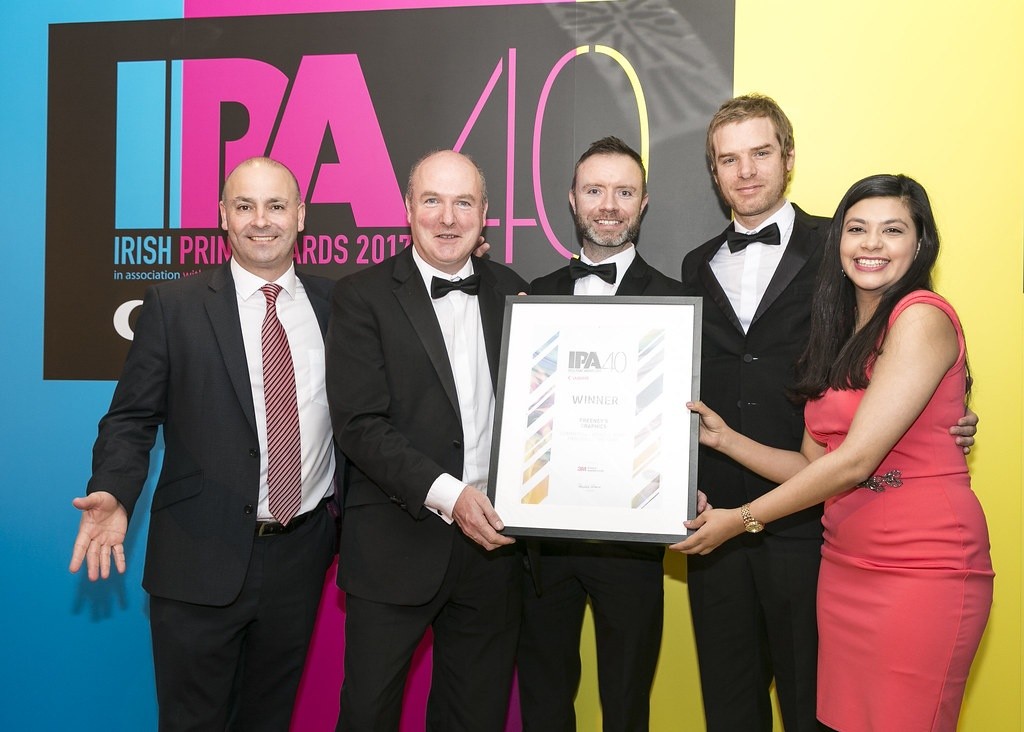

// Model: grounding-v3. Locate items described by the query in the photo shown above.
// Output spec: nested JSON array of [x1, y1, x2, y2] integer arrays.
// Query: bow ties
[[569, 257, 617, 285], [431, 273, 480, 299], [726, 222, 780, 254]]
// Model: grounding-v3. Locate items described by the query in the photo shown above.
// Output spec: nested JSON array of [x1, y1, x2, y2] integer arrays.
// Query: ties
[[258, 283, 302, 526]]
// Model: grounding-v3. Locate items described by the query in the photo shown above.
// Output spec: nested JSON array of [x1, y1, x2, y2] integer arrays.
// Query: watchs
[[741, 502, 764, 534]]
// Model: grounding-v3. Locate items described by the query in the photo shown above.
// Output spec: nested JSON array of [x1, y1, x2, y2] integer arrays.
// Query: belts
[[254, 494, 335, 537]]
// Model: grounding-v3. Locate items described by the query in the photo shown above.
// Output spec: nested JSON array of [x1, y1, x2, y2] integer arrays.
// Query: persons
[[681, 93, 979, 732], [519, 136, 712, 732], [72, 155, 491, 731], [326, 148, 531, 731], [669, 174, 994, 732]]
